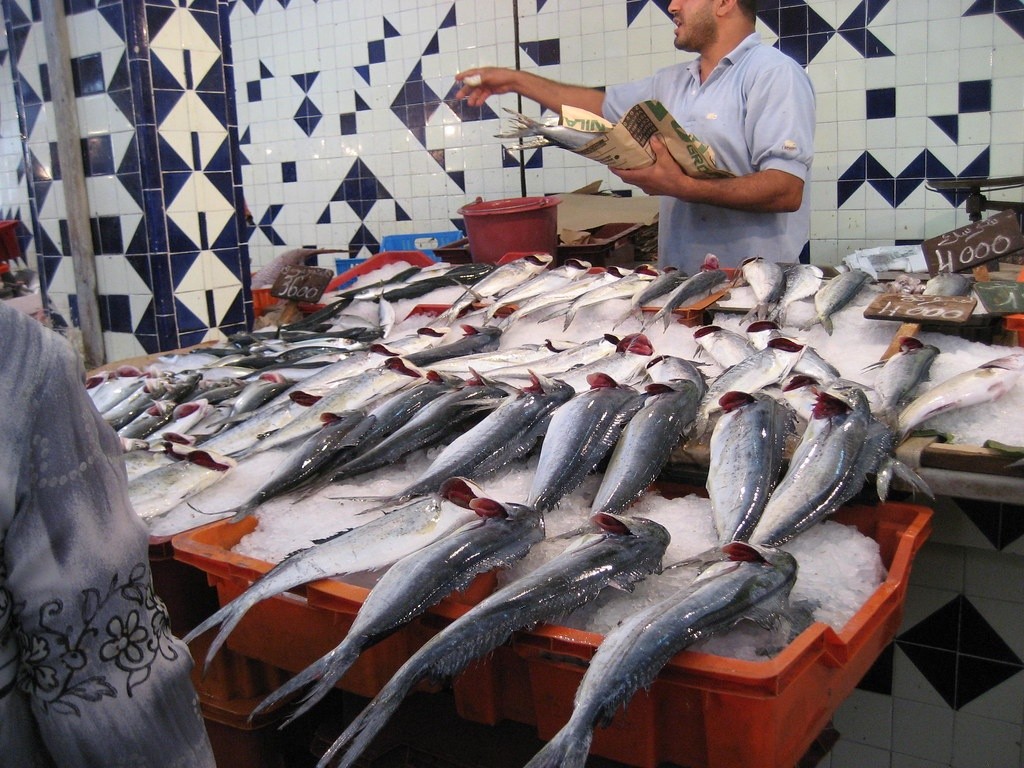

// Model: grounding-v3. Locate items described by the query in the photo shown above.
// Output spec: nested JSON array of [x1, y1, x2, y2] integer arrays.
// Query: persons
[[0, 305, 217, 768], [457, 0, 816, 272]]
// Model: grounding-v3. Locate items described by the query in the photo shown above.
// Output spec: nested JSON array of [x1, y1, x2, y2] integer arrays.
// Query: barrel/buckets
[[457, 195, 563, 271]]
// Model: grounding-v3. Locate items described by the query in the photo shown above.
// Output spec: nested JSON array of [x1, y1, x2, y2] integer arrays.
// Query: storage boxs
[[309, 694, 842, 768], [251, 271, 276, 317], [174, 619, 341, 768], [0, 219, 22, 261], [432, 221, 644, 271], [430, 499, 936, 768], [334, 230, 464, 290], [168, 511, 444, 700], [298, 250, 435, 317]]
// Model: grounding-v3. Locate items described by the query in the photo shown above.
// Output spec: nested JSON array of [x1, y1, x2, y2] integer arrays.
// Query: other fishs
[[80, 245, 1024, 768], [492, 105, 601, 151]]
